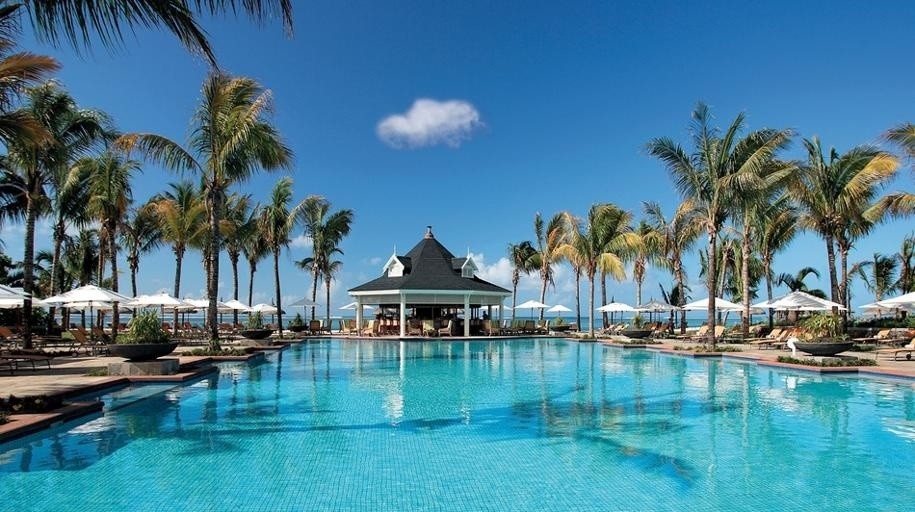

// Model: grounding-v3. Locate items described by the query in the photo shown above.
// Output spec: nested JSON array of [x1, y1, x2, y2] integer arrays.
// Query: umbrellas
[[287, 297, 323, 326], [338, 302, 375, 322], [546, 304, 574, 318], [511, 298, 553, 321], [480, 305, 516, 320], [0, 283, 285, 340]]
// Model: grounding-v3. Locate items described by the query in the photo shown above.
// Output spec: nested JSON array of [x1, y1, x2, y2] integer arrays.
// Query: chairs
[[0, 318, 278, 375], [305, 311, 549, 337]]
